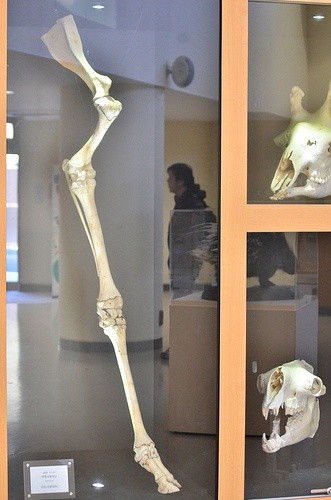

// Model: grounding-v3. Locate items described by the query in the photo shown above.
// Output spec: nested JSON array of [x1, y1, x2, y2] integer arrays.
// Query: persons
[[161, 162, 217, 361], [257, 233, 287, 286]]
